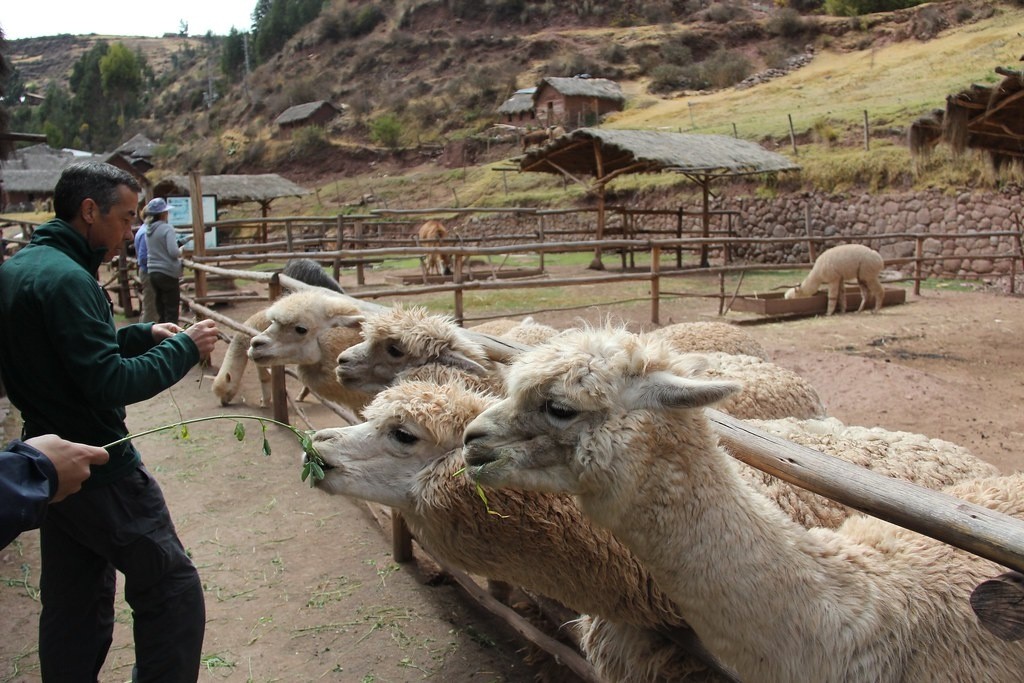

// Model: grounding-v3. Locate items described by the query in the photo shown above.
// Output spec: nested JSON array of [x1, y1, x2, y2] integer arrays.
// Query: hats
[[143, 198, 175, 212]]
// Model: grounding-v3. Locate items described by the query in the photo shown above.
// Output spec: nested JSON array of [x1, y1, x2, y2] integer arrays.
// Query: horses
[[419, 219, 452, 275]]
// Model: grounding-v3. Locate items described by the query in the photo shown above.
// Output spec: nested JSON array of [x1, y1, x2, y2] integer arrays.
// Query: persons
[[132, 205, 159, 323], [0, 434, 111, 548], [141, 196, 193, 325], [0, 160, 219, 683]]
